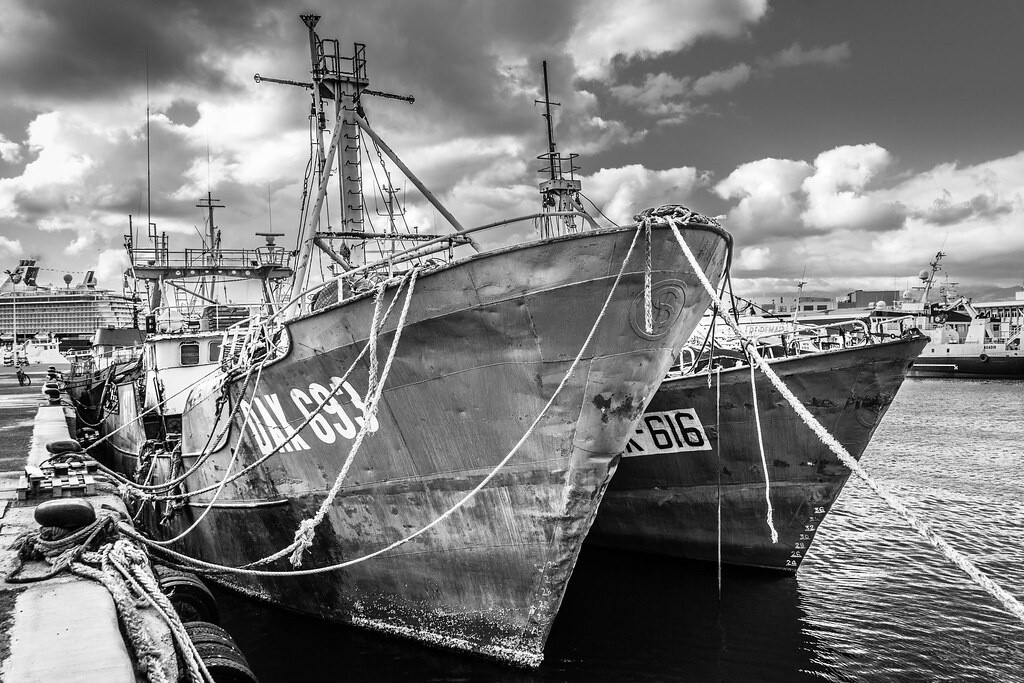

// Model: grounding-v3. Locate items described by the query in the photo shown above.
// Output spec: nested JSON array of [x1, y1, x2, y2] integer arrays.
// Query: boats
[[68, 15, 734, 672], [685, 238, 1024, 382], [533, 56, 929, 574]]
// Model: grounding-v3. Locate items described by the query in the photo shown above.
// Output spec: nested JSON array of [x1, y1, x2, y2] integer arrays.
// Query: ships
[[0, 255, 161, 364]]
